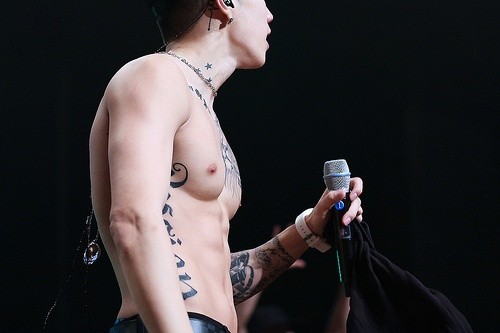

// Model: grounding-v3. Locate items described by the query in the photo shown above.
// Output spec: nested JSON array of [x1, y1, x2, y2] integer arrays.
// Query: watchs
[[295, 207, 332, 254]]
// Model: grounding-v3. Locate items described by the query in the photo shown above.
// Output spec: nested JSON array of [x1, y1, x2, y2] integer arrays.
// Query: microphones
[[322, 159, 355, 297]]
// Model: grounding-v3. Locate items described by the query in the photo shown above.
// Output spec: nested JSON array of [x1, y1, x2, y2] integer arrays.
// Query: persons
[[246, 280, 351, 333], [90, 0, 363, 333]]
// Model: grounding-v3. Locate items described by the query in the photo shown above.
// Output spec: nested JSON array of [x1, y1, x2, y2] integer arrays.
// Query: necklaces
[[163, 49, 219, 99]]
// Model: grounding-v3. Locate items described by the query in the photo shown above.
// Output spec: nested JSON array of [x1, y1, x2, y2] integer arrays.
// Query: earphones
[[223, 0, 234, 8]]
